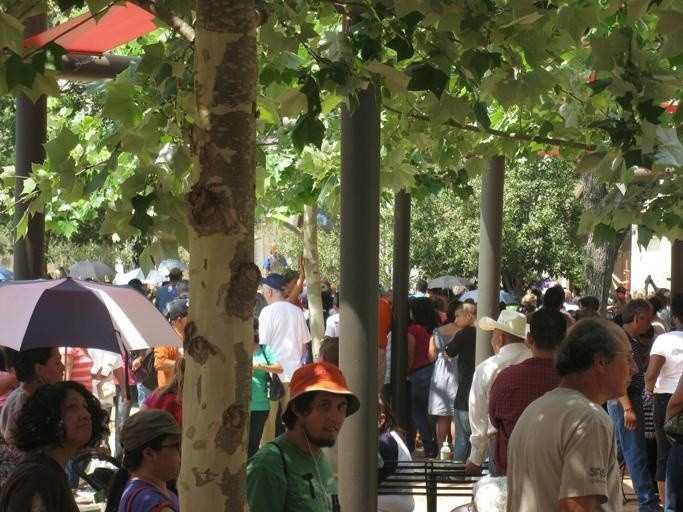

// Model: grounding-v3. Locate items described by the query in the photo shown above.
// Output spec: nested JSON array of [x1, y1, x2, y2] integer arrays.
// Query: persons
[[0, 268, 191, 512], [247, 245, 683, 512]]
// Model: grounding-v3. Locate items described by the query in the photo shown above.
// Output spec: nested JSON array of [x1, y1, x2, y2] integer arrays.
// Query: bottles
[[440, 442, 451, 463]]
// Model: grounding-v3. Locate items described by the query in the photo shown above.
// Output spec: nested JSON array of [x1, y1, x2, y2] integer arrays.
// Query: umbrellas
[[68, 259, 186, 286]]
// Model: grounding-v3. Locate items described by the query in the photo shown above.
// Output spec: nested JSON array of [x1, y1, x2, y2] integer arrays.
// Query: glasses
[[157, 441, 181, 452], [615, 350, 635, 361]]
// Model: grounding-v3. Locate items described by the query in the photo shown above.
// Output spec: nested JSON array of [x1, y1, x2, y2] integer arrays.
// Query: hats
[[259, 273, 286, 292], [162, 298, 189, 321], [477, 309, 532, 341], [278, 362, 361, 431], [116, 408, 182, 454]]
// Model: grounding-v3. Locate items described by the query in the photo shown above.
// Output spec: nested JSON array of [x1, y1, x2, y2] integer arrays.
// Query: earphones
[[299, 405, 305, 417]]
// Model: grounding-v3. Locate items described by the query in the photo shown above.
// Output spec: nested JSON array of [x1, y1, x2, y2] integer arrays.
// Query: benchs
[[377, 460, 489, 512]]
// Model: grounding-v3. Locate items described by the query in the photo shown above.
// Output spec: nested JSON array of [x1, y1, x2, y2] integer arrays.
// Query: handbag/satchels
[[131, 351, 158, 392]]
